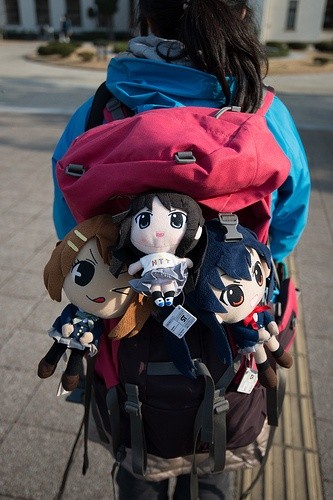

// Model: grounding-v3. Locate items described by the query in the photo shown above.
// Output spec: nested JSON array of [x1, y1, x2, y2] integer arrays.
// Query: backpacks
[[55, 79, 300, 500]]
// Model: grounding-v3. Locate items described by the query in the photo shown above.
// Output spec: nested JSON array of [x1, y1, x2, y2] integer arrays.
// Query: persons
[[52, 0, 311, 500]]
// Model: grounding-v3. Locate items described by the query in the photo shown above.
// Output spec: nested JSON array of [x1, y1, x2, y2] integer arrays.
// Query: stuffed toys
[[38, 190, 294, 391]]
